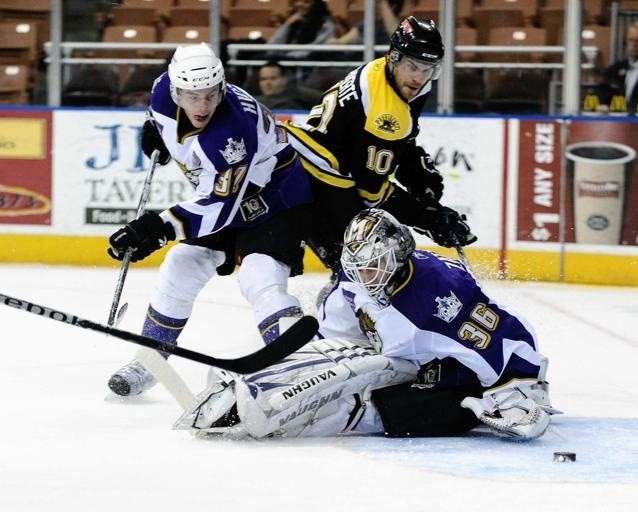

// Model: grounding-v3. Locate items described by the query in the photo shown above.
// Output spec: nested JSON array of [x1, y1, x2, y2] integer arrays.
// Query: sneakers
[[108, 361, 157, 395]]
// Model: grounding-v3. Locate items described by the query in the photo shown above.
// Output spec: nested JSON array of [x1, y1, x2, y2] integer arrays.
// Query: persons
[[108, 42, 313, 395], [238, 0, 404, 108], [284, 16, 478, 307], [581, 20, 637, 114], [173, 206, 563, 441]]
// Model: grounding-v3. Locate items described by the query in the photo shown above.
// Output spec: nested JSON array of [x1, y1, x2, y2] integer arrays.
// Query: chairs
[[0, 0, 638, 113]]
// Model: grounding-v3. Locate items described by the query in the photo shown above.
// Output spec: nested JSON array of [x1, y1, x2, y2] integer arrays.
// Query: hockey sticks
[[0, 294, 319, 375], [107, 150, 160, 329]]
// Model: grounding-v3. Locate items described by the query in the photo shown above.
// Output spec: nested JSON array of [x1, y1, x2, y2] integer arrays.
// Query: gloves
[[108, 211, 176, 262], [397, 154, 444, 202], [142, 120, 171, 165], [414, 209, 477, 247]]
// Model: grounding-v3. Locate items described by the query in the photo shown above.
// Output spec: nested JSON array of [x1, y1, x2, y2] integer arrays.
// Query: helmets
[[168, 42, 226, 106], [340, 208, 416, 296], [390, 16, 445, 80]]
